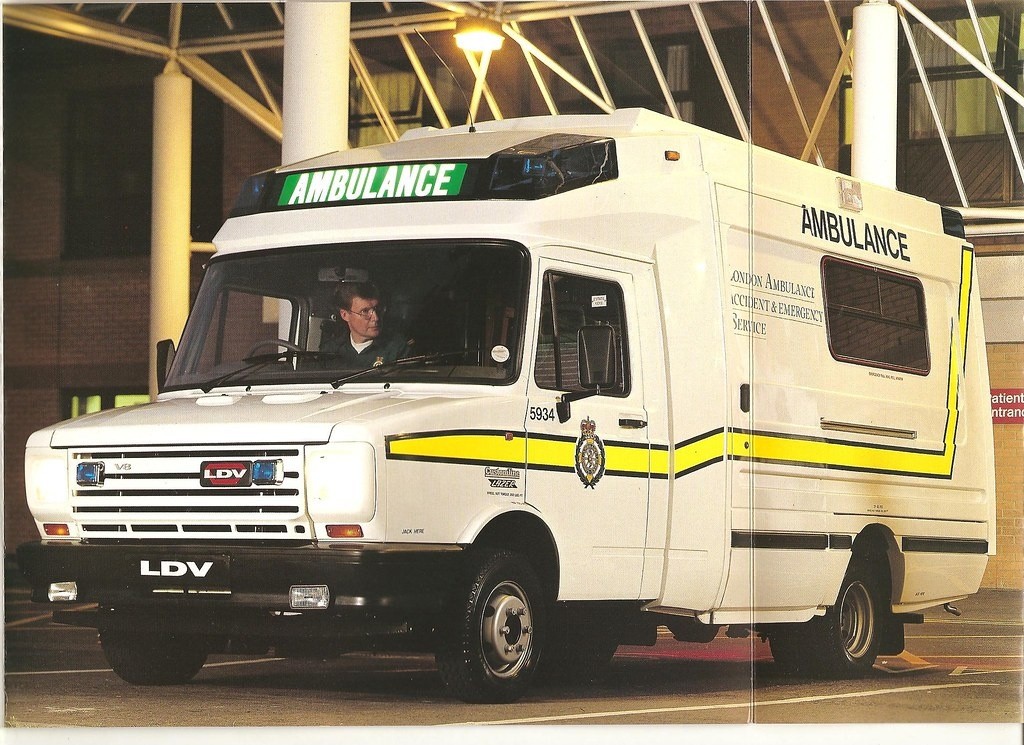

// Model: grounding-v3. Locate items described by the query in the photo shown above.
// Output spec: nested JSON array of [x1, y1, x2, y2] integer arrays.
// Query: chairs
[[529, 300, 589, 393]]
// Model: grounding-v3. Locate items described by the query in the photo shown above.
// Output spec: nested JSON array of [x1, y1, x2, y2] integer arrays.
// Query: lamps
[[452, 10, 505, 51]]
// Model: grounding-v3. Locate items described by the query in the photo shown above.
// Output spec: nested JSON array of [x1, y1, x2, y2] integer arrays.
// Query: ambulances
[[23, 108, 996, 703]]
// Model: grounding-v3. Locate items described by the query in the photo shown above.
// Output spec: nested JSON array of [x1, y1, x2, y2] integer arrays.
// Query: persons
[[316, 281, 415, 369]]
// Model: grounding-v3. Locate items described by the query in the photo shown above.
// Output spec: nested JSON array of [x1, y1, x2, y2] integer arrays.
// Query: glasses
[[343, 303, 386, 320]]
